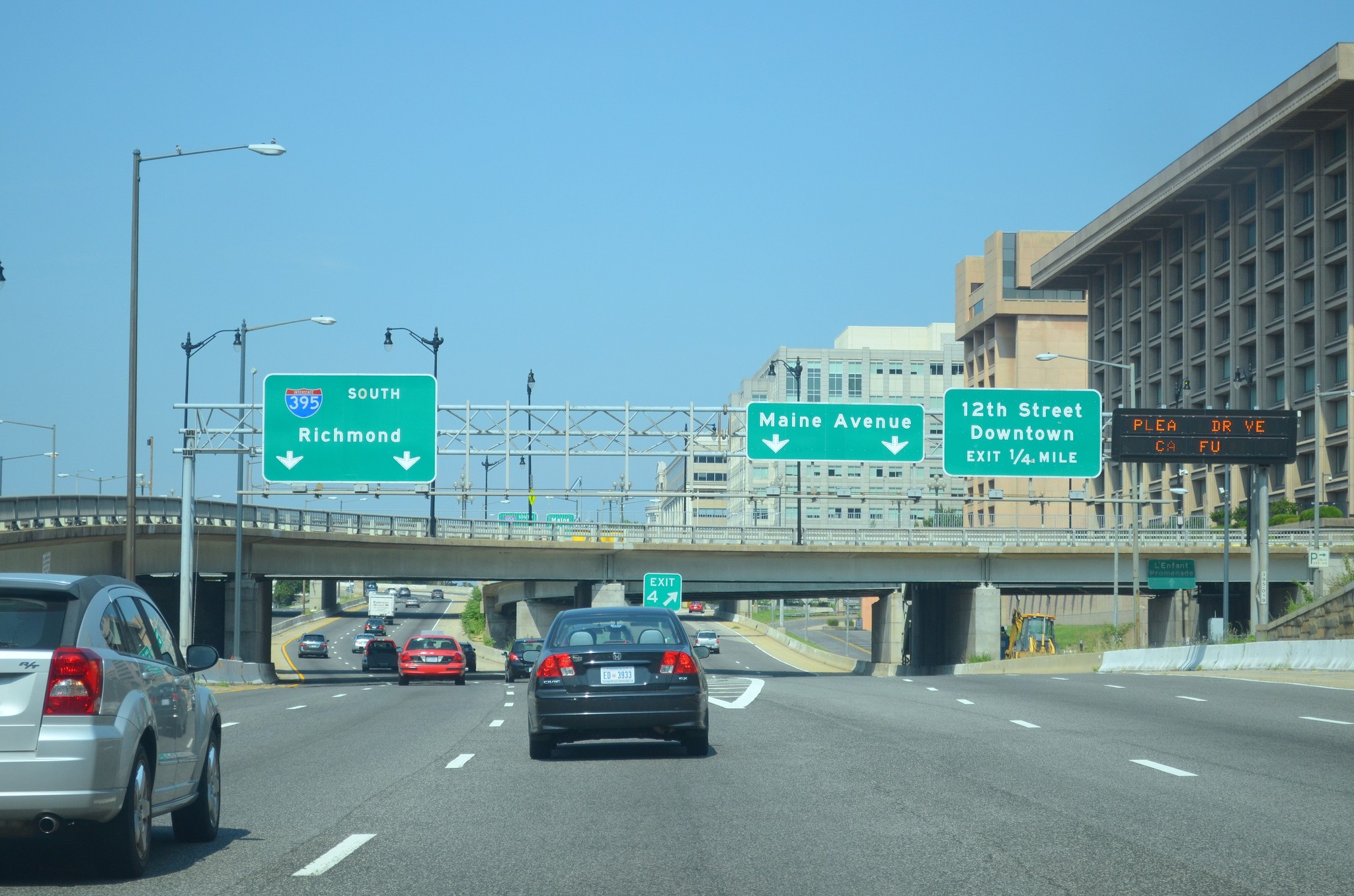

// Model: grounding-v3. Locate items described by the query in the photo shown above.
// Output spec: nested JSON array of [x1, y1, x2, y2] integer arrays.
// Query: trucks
[[368, 595, 398, 625]]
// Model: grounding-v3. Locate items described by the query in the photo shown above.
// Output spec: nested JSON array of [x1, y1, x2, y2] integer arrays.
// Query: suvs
[[693, 629, 721, 654], [365, 582, 378, 591]]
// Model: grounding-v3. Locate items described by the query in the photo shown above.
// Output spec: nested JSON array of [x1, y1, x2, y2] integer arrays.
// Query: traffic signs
[[745, 401, 926, 463], [264, 373, 436, 482], [643, 573, 683, 611], [1147, 559, 1197, 590]]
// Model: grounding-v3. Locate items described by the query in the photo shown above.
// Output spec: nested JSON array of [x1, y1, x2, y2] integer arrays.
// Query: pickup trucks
[[361, 639, 400, 673]]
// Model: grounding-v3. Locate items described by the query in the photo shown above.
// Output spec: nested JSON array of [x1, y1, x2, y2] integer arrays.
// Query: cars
[[351, 618, 387, 652], [296, 633, 330, 660], [365, 589, 378, 596], [687, 601, 705, 614], [0, 572, 225, 883], [430, 589, 445, 599], [383, 587, 421, 608], [345, 584, 354, 593], [399, 634, 477, 686], [501, 606, 711, 759]]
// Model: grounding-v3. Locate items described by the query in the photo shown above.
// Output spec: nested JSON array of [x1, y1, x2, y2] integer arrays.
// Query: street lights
[[452, 466, 475, 519], [123, 140, 287, 586], [595, 474, 662, 529], [383, 324, 445, 537], [1232, 363, 1255, 547], [0, 451, 145, 495], [526, 368, 537, 524], [480, 452, 528, 526], [178, 315, 336, 663], [1034, 351, 1142, 650], [544, 495, 579, 523], [766, 356, 804, 546], [1174, 376, 1193, 535], [683, 422, 718, 533]]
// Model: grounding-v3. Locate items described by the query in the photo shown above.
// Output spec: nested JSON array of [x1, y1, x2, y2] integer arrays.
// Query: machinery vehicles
[[1002, 608, 1060, 660]]
